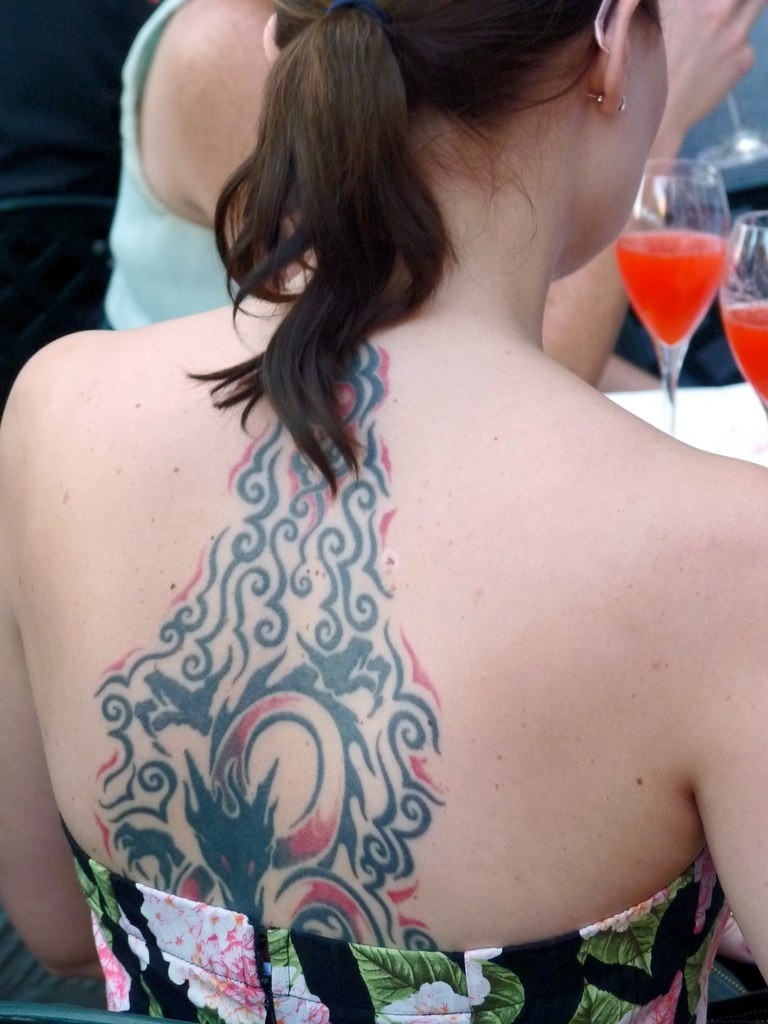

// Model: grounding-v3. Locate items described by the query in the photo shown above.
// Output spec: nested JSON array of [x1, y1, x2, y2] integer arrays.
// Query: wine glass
[[617, 157, 729, 436], [696, 89, 768, 165]]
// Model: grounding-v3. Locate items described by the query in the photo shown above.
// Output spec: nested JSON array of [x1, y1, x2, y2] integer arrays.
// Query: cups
[[717, 209, 768, 420]]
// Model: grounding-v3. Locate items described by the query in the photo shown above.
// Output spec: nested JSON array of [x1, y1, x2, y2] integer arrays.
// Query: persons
[[101, 1, 761, 390], [1, 2, 768, 1024]]
